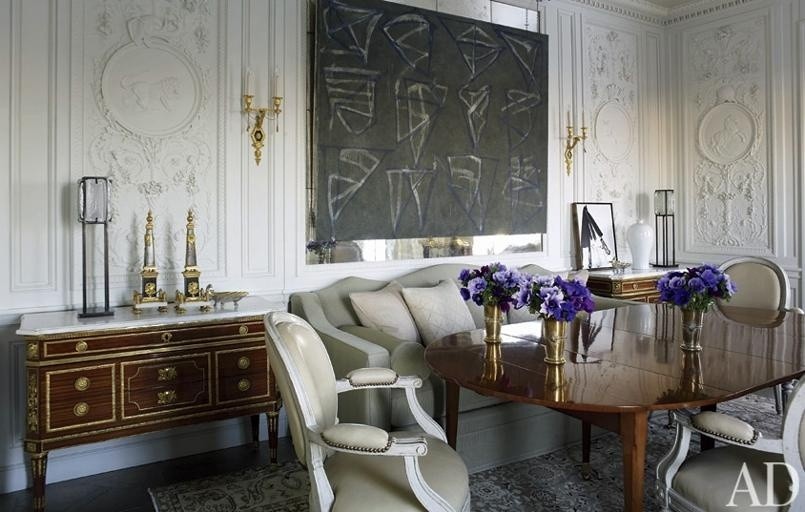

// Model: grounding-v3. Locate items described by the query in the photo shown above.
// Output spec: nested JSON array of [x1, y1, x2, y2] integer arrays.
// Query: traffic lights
[[508, 270, 589, 323], [402, 278, 476, 346], [337, 325, 430, 383], [349, 279, 422, 344]]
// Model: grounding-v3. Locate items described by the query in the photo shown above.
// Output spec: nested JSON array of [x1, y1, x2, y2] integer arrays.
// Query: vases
[[468, 374, 535, 399], [459, 261, 519, 314], [657, 261, 738, 313], [654, 388, 711, 404], [305, 240, 337, 254]]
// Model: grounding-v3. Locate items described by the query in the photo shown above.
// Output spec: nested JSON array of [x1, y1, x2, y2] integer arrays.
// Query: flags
[[318, 249, 332, 264], [483, 304, 506, 344], [627, 219, 654, 270], [482, 343, 504, 384], [540, 316, 568, 366], [679, 350, 704, 393], [544, 364, 565, 403], [678, 306, 710, 352]]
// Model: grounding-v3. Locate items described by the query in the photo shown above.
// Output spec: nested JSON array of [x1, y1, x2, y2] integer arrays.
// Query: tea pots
[[573, 309, 617, 364], [572, 202, 618, 272]]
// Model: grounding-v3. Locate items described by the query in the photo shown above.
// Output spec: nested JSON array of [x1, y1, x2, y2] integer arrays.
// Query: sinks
[[289, 264, 650, 474]]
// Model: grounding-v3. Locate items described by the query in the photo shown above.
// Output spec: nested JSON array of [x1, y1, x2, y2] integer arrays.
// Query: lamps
[[264, 311, 471, 511], [714, 255, 717, 415], [655, 374, 805, 512]]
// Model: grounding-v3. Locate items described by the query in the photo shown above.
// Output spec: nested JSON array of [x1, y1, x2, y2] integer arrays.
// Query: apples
[[582, 111, 585, 127], [568, 109, 572, 128], [274, 73, 279, 97], [247, 70, 253, 95]]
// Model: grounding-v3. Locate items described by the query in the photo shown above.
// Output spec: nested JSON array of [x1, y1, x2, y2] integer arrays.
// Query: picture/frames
[[578, 262, 705, 303], [15, 297, 283, 512], [424, 304, 805, 511]]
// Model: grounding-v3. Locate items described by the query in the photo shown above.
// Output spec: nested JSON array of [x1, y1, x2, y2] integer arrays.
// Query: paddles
[[147, 387, 805, 512]]
